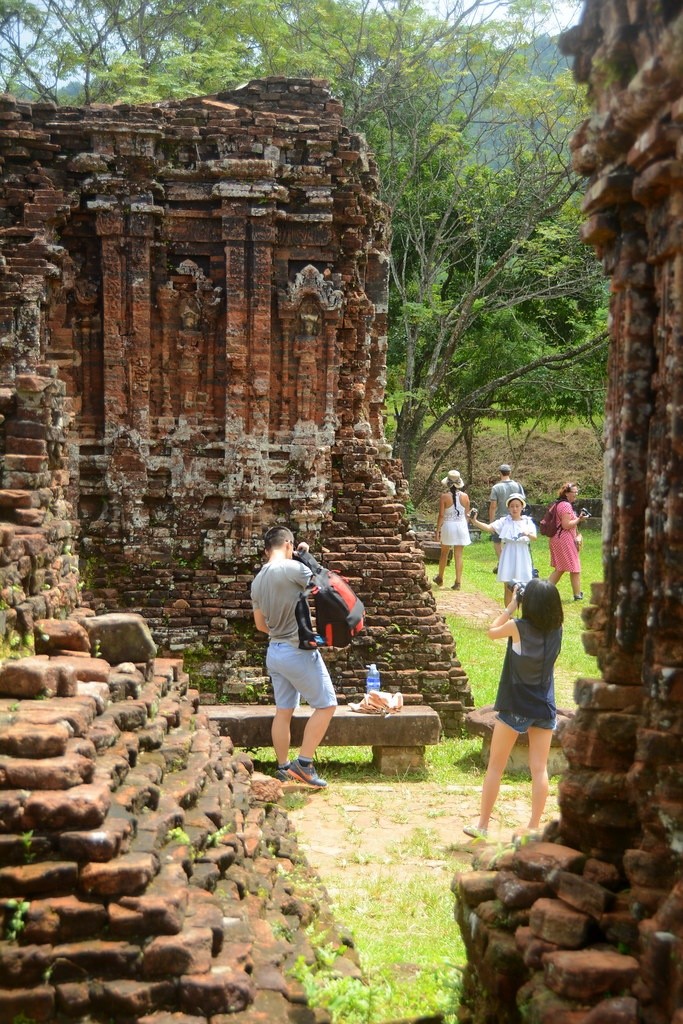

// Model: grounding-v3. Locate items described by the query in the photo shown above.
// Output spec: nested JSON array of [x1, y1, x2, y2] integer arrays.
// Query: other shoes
[[463, 824, 488, 839], [433, 575, 443, 586], [451, 582, 461, 591], [573, 592, 583, 601]]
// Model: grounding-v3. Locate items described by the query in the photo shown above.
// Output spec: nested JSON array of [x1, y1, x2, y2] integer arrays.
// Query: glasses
[[569, 490, 578, 495]]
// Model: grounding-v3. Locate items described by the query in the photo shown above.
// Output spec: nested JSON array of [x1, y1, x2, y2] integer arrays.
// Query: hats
[[506, 492, 527, 509], [497, 464, 511, 472], [440, 469, 465, 489]]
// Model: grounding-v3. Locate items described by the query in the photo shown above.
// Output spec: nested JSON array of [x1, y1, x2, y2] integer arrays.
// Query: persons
[[468, 493, 537, 608], [250, 525, 338, 787], [489, 464, 526, 573], [433, 470, 470, 590], [549, 483, 588, 600], [464, 577, 564, 838]]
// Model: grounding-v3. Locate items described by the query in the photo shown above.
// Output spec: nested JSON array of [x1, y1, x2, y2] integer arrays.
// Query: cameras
[[581, 508, 592, 518], [507, 580, 527, 601]]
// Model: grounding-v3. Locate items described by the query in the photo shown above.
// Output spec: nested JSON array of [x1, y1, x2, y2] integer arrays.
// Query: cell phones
[[470, 508, 477, 518]]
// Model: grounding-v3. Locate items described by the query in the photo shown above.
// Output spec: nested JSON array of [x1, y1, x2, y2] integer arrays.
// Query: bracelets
[[505, 608, 511, 616]]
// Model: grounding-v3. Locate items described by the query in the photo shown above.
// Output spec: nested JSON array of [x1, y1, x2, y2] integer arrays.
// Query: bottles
[[367, 663, 380, 693]]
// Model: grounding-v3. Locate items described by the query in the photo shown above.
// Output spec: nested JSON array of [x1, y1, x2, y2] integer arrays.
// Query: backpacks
[[295, 555, 367, 651], [539, 498, 570, 538]]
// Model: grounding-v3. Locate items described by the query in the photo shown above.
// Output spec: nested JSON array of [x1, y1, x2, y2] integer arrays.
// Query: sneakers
[[286, 758, 328, 788], [275, 769, 300, 782]]
